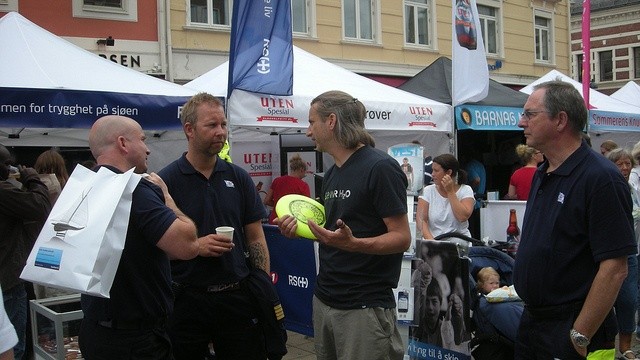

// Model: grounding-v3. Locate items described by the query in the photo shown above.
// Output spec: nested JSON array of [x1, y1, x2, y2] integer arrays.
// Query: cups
[[216, 226, 234, 244]]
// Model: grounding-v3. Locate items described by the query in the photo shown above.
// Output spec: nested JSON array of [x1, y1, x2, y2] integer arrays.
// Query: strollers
[[434, 232, 524, 360]]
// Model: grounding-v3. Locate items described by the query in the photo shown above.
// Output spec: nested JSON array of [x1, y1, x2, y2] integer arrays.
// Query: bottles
[[506, 210, 521, 258]]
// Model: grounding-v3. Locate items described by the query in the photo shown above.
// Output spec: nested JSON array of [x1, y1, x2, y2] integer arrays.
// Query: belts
[[523, 303, 583, 317], [192, 281, 242, 292], [96, 319, 168, 330]]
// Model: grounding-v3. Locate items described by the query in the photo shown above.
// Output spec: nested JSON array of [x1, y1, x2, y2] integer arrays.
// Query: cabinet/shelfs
[[28, 294, 85, 358]]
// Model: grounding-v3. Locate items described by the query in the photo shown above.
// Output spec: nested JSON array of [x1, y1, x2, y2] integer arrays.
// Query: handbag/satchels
[[18, 161, 150, 298]]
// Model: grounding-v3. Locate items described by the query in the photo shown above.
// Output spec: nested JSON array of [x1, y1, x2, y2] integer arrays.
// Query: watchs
[[570, 328, 591, 348]]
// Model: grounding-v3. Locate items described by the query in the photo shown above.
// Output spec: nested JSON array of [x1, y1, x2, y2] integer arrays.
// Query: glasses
[[520, 110, 556, 119]]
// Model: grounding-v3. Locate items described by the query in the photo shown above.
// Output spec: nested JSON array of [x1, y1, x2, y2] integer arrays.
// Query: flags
[[451, 0, 491, 108], [228, 0, 295, 97]]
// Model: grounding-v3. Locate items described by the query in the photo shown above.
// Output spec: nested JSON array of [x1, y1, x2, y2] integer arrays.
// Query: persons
[[599, 140, 617, 156], [412, 239, 471, 357], [416, 154, 477, 249], [399, 157, 415, 191], [602, 147, 639, 359], [1, 285, 20, 360], [262, 157, 312, 225], [506, 143, 545, 200], [462, 145, 487, 239], [476, 265, 502, 296], [272, 90, 412, 360], [627, 141, 639, 254], [33, 150, 67, 203], [511, 82, 636, 360], [156, 92, 287, 360], [79, 114, 201, 360], [1, 141, 55, 360]]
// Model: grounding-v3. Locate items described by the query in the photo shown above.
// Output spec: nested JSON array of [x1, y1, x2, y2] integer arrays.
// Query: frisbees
[[275, 194, 326, 239]]
